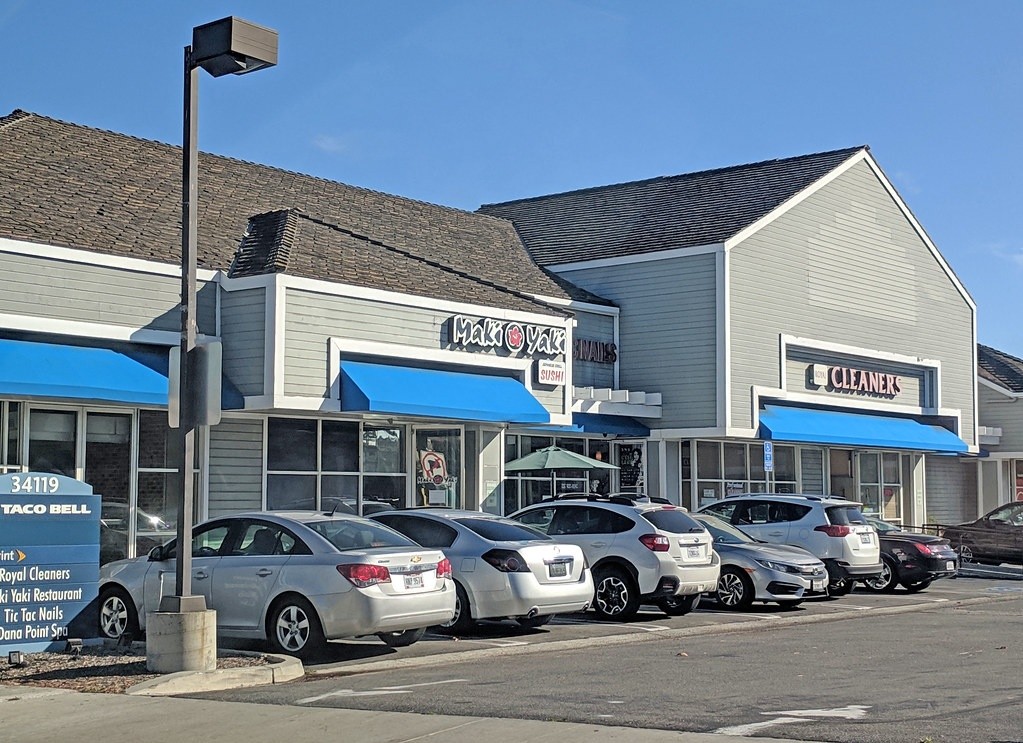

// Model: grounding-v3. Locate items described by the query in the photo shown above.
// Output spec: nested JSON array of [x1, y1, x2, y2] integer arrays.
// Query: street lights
[[147, 16, 277, 677]]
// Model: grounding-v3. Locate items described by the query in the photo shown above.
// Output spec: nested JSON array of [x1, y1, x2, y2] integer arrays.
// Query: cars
[[100, 511, 454, 664], [329, 509, 594, 636], [100, 498, 167, 560], [578, 513, 830, 611], [859, 519, 962, 594], [941, 501, 1023, 566]]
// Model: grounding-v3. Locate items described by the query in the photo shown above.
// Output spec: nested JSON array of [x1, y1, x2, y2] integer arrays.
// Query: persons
[[631, 448, 642, 485]]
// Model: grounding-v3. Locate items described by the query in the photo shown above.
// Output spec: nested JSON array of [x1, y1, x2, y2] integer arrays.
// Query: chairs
[[352, 531, 374, 548], [247, 531, 284, 554]]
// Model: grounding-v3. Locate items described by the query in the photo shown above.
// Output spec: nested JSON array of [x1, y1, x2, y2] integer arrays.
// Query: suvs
[[284, 494, 397, 519], [690, 493, 884, 596], [504, 493, 721, 622]]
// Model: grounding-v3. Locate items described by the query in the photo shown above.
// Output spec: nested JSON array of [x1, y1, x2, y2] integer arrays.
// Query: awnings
[[758, 403, 969, 452], [339, 359, 550, 423], [0, 333, 245, 409]]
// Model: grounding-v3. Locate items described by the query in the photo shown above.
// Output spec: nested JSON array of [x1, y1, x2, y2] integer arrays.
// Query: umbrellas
[[505, 444, 621, 496]]
